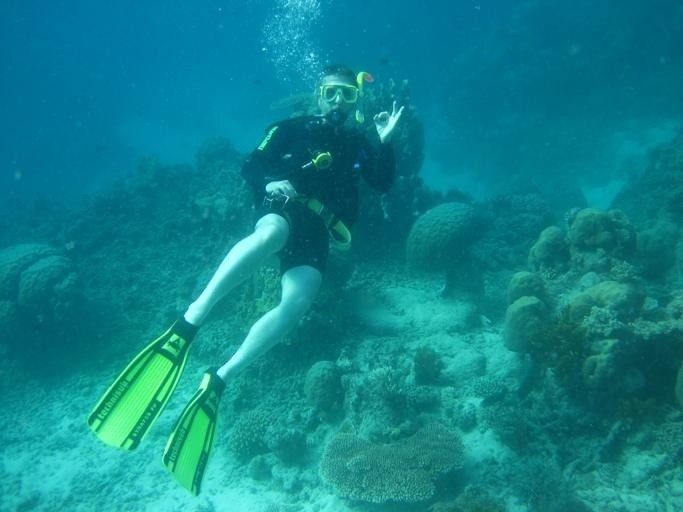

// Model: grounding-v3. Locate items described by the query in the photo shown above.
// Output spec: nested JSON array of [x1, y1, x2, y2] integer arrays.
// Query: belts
[[296, 193, 351, 252]]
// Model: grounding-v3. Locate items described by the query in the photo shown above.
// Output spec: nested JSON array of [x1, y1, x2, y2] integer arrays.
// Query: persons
[[88, 62, 405, 495]]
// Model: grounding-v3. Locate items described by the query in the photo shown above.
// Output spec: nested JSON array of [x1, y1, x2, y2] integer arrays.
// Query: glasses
[[320, 83, 360, 105]]
